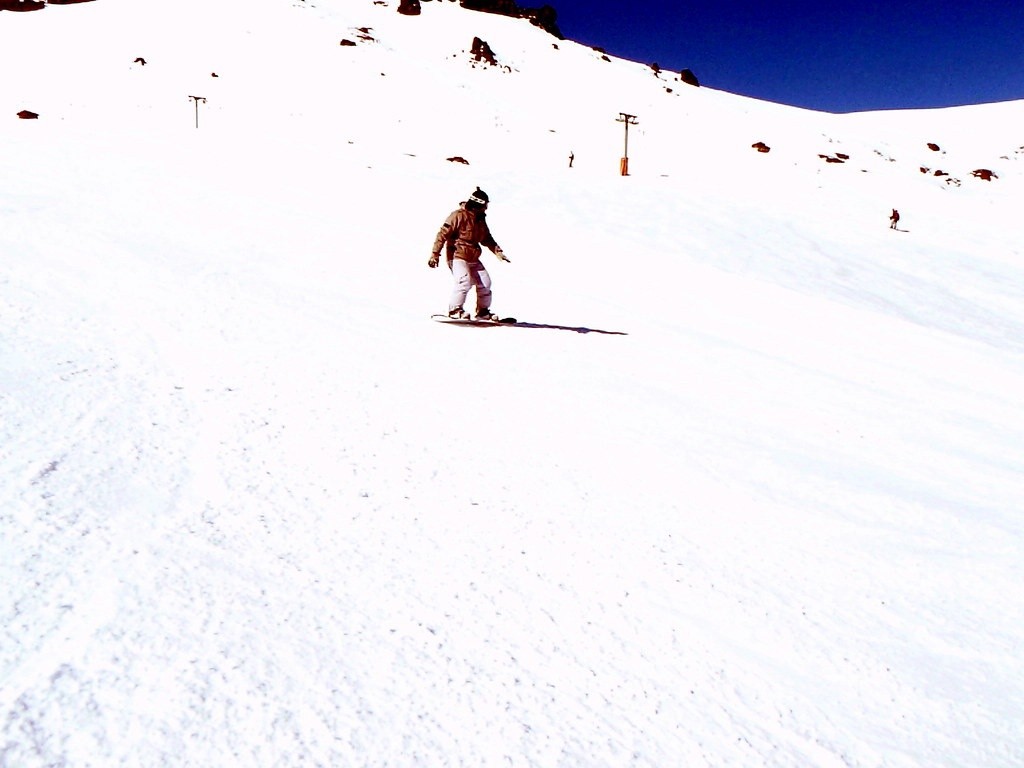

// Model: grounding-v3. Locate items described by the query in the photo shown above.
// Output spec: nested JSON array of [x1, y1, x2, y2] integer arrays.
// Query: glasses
[[485, 201, 488, 208]]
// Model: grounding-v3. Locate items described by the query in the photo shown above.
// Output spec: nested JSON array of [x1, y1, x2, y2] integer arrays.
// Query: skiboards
[[430, 313, 630, 337]]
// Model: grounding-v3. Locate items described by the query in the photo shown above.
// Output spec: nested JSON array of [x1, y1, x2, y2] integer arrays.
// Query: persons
[[890, 210, 899, 229], [428, 186, 511, 320]]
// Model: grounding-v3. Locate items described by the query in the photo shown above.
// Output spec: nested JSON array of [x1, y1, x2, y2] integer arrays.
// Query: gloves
[[497, 252, 510, 263], [428, 252, 439, 268]]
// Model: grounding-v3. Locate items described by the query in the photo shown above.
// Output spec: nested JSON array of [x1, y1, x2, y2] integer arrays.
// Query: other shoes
[[476, 309, 498, 321], [449, 308, 470, 319]]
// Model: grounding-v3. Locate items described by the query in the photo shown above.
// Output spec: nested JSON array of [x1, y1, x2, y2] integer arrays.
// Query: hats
[[469, 186, 488, 207]]
